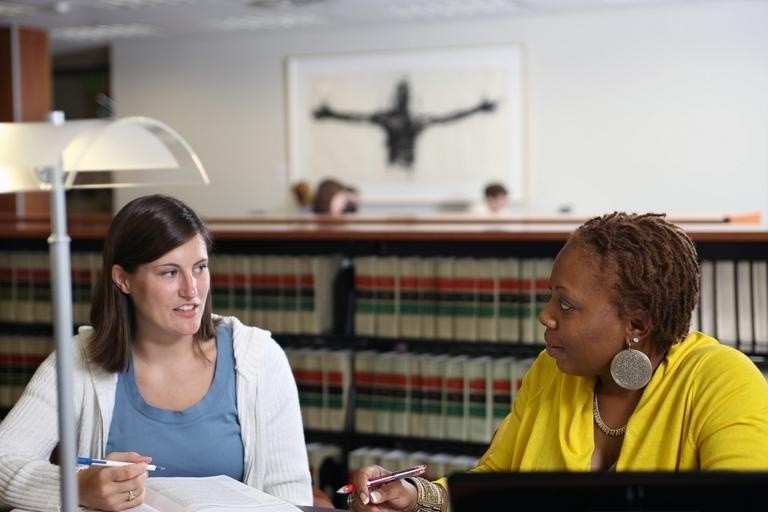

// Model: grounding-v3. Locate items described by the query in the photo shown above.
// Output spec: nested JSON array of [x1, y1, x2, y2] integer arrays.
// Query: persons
[[342, 187, 363, 212], [292, 180, 315, 216], [482, 184, 511, 214], [344, 211, 766, 511], [306, 181, 346, 214], [312, 82, 497, 166], [2, 194, 317, 512]]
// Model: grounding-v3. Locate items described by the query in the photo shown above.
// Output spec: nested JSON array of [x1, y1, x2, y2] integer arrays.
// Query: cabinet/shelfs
[[0, 209, 768, 511]]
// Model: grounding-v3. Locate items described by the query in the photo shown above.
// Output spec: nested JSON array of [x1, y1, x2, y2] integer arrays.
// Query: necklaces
[[590, 380, 628, 437]]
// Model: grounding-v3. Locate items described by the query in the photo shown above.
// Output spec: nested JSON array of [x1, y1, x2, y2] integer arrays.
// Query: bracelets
[[409, 472, 448, 512]]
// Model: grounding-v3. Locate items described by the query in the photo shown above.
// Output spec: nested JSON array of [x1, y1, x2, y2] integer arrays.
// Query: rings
[[128, 490, 134, 501], [347, 493, 356, 504]]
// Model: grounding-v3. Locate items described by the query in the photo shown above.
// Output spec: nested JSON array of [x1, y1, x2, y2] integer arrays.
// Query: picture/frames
[[284, 40, 530, 215]]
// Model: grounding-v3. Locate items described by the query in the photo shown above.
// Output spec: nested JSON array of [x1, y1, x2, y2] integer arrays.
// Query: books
[[120, 471, 305, 512]]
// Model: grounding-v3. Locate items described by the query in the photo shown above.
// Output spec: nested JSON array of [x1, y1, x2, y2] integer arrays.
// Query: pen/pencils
[[336, 465, 429, 494], [77, 457, 166, 471]]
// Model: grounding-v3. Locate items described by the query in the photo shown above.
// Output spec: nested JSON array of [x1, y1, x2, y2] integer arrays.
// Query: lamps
[[0, 108, 216, 512]]
